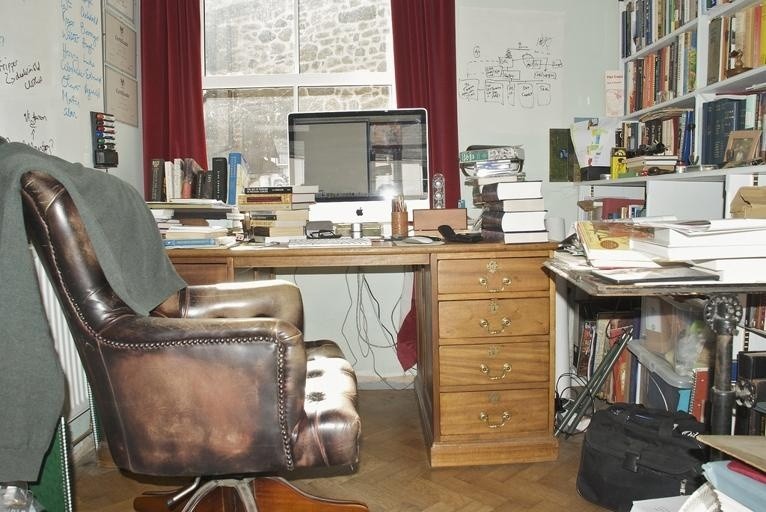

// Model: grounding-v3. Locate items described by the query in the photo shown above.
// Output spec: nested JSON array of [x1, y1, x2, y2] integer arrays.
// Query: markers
[[96, 114, 116, 149]]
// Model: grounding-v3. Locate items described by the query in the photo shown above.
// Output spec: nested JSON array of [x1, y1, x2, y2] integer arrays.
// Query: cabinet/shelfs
[[617, 0, 766, 176], [575, 176, 725, 403]]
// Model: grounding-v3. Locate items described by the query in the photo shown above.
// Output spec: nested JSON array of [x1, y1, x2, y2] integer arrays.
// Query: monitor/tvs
[[286, 105, 430, 239]]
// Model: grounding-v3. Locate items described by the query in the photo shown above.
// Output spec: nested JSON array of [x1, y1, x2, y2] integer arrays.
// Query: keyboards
[[286, 238, 373, 247]]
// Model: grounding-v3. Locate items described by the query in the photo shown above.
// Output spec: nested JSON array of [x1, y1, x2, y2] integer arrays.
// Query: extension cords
[[555, 406, 590, 433]]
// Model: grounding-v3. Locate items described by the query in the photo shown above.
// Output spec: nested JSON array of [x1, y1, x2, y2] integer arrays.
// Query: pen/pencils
[[392, 195, 407, 212], [372, 239, 402, 241]]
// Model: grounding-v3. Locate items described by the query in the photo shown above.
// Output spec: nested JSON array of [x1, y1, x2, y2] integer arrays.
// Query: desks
[[544, 257, 766, 464], [156, 240, 558, 468]]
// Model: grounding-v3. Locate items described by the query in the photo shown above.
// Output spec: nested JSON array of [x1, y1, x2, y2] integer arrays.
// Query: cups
[[391, 212, 409, 238]]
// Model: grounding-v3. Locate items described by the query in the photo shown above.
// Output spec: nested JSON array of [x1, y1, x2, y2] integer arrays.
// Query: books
[[734, 350, 766, 433], [145, 150, 318, 247], [481, 179, 550, 244], [551, 216, 766, 283], [745, 292, 766, 331], [568, 286, 746, 423], [627, 433, 766, 511], [603, 1, 766, 176]]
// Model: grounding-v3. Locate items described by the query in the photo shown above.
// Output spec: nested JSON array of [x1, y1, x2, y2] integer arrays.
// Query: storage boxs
[[625, 295, 706, 413]]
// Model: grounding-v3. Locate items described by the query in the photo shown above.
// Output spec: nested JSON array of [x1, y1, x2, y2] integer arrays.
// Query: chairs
[[18, 170, 369, 511]]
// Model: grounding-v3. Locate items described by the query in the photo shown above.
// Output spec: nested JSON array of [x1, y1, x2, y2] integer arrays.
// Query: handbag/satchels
[[573, 401, 719, 511]]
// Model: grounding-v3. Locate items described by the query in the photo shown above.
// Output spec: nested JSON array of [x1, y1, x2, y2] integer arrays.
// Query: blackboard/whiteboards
[[454, 0, 620, 226], [0, 0, 108, 178]]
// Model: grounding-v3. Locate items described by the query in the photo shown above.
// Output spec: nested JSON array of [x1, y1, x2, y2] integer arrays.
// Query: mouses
[[403, 236, 433, 243]]
